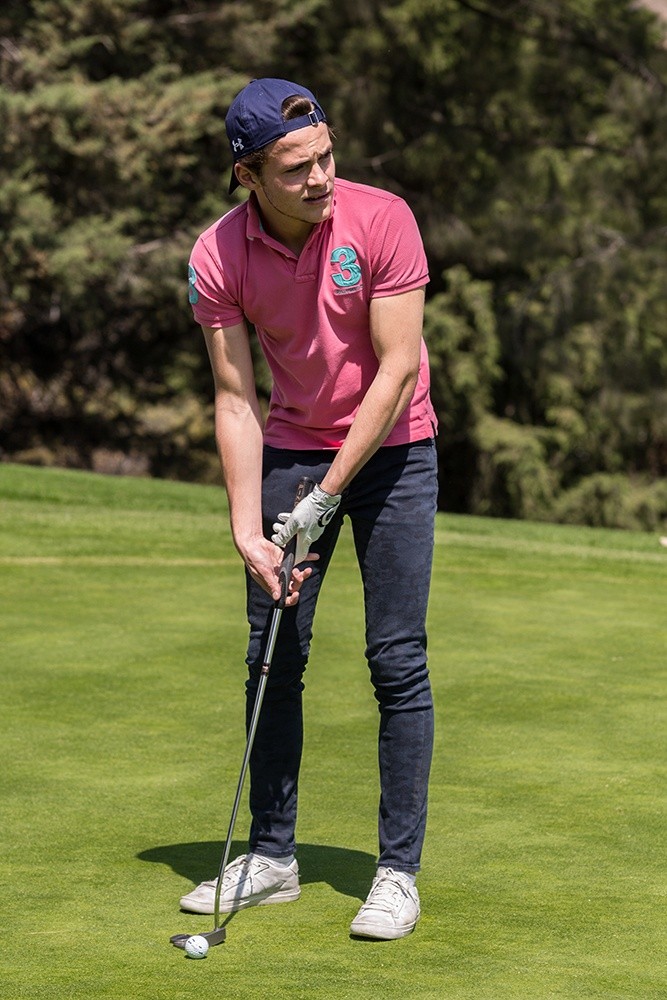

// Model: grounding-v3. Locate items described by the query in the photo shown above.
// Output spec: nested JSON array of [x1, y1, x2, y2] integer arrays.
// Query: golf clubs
[[168, 475, 316, 950]]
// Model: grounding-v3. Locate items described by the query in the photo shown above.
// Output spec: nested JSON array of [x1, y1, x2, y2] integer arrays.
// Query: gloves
[[271, 483, 342, 568]]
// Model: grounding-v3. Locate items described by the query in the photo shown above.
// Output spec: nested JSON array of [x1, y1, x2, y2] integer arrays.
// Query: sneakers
[[180, 854, 301, 914], [350, 866, 420, 939]]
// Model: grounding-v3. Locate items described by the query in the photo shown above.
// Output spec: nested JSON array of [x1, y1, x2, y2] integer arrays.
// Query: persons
[[178, 78, 440, 938]]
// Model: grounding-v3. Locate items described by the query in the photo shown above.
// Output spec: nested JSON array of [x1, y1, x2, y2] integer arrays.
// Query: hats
[[225, 78, 327, 196]]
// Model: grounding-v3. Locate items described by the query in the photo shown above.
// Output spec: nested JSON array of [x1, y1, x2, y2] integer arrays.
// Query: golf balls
[[184, 935, 209, 959]]
